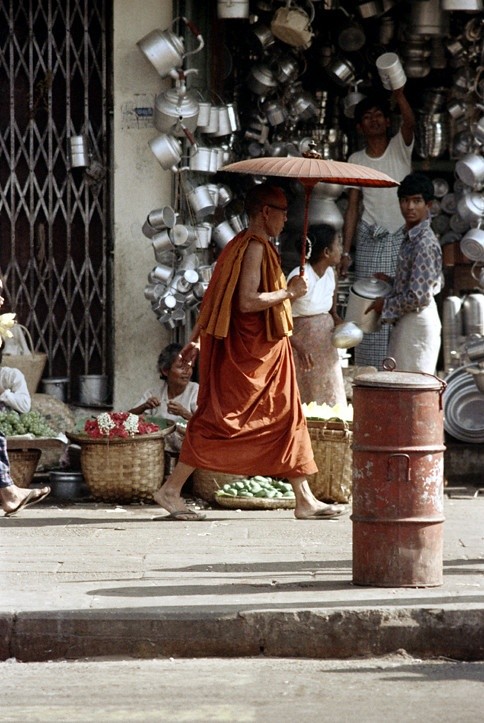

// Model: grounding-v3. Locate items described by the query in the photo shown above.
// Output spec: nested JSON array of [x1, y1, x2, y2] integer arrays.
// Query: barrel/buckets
[[45, 374, 110, 410]]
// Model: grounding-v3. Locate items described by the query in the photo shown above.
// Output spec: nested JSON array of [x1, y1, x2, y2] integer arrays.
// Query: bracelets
[[342, 252, 353, 256]]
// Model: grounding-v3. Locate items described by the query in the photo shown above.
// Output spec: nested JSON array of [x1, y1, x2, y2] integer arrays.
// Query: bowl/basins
[[443, 362, 484, 443]]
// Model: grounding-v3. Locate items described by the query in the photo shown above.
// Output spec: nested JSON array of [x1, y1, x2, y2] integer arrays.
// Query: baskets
[[193, 468, 248, 504], [79, 438, 165, 503], [7, 447, 44, 487], [304, 419, 355, 504]]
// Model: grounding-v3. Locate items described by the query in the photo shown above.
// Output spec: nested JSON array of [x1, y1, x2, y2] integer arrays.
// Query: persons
[[0, 432, 52, 517], [284, 221, 351, 412], [1, 338, 33, 415], [362, 169, 446, 372], [150, 184, 353, 524], [336, 84, 417, 375], [126, 342, 204, 475]]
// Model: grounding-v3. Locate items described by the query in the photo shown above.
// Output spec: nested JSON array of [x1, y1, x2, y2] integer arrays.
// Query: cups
[[67, 129, 93, 169], [140, 204, 212, 331], [190, 81, 237, 216], [214, 0, 482, 296]]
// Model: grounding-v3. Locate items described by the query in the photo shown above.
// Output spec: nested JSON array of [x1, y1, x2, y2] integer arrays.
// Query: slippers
[[299, 505, 348, 520], [4, 486, 51, 518], [152, 510, 206, 522]]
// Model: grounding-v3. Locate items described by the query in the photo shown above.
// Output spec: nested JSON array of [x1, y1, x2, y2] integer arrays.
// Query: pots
[[345, 276, 390, 334], [48, 470, 84, 498]]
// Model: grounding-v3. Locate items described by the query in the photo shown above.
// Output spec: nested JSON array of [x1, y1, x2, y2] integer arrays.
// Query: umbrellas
[[217, 156, 402, 278]]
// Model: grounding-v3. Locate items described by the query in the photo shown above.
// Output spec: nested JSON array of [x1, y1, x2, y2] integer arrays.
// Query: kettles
[[148, 123, 198, 173], [137, 15, 205, 79], [152, 69, 201, 137]]
